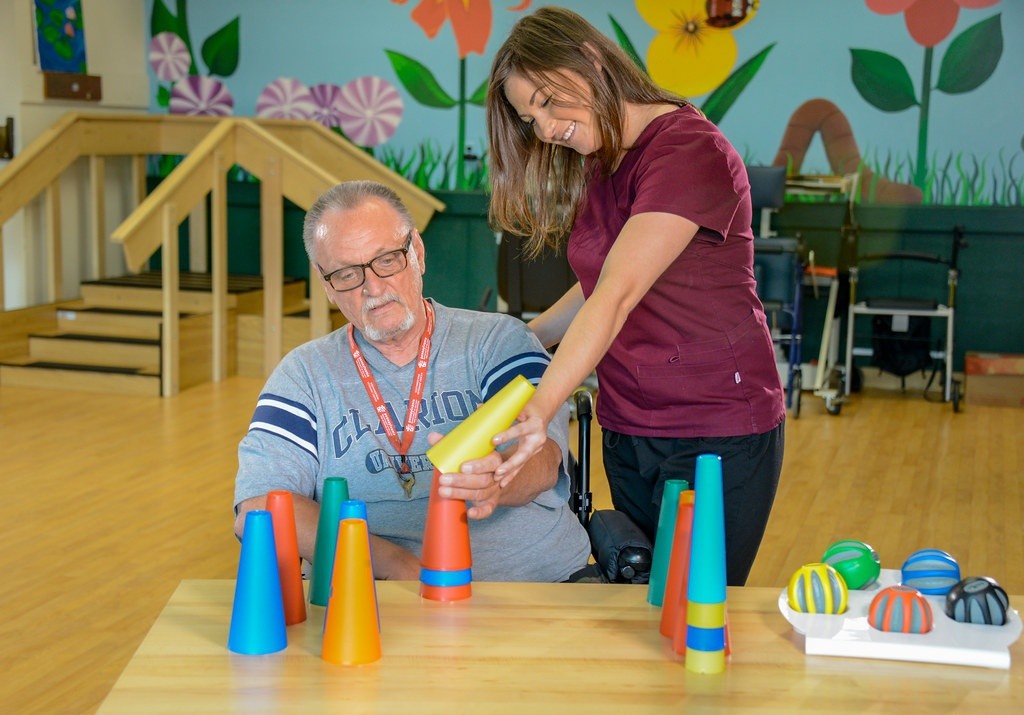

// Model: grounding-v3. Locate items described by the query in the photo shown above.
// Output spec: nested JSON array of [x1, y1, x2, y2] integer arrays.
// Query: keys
[[400, 476, 415, 498]]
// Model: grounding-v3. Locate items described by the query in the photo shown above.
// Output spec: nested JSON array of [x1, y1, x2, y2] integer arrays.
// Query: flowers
[[33, 0, 1007, 147]]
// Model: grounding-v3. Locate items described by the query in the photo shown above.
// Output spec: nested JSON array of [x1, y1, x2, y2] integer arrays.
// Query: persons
[[457, 2, 785, 600], [197, 184, 596, 594]]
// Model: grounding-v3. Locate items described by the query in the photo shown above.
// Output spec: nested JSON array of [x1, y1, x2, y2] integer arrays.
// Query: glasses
[[318, 231, 412, 293]]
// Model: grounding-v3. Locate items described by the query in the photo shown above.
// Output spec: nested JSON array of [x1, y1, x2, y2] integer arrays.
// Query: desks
[[93, 577, 1023, 715]]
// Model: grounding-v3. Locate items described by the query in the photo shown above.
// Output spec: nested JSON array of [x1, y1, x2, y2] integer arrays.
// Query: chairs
[[564, 391, 652, 584]]
